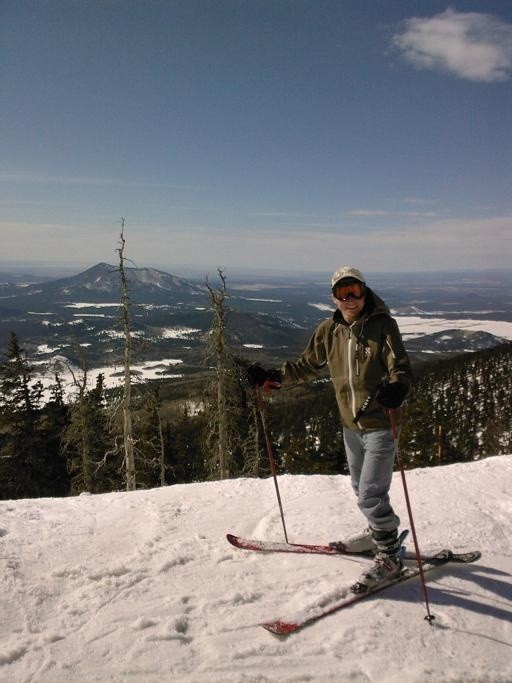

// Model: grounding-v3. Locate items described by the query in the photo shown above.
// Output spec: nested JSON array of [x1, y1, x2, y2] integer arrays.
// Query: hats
[[331, 268, 364, 289]]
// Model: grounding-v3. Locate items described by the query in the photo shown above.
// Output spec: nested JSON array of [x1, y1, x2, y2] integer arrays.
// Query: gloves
[[247, 366, 268, 386], [372, 382, 404, 408]]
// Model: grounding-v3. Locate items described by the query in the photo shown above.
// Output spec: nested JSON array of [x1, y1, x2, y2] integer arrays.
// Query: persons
[[242, 265, 415, 597]]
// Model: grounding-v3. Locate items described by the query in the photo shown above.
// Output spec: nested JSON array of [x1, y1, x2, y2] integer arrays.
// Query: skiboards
[[225, 535, 481, 637]]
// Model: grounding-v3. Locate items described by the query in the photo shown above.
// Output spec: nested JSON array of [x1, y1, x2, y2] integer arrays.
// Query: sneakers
[[344, 528, 377, 552], [359, 554, 400, 584]]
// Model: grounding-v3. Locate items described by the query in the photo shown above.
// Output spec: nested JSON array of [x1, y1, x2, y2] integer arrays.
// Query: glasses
[[332, 281, 365, 300]]
